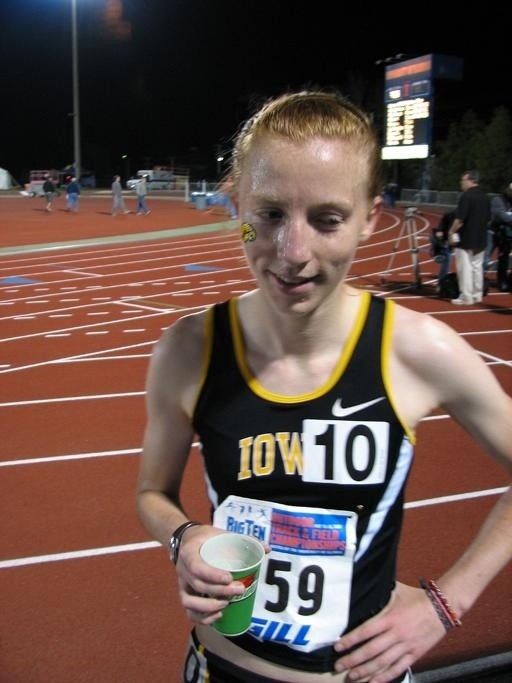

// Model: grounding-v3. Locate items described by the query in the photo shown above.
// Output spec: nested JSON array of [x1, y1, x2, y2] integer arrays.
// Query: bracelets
[[419, 574, 465, 633]]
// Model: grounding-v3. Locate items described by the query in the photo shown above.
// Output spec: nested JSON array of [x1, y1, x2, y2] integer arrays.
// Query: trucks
[[126, 170, 175, 191]]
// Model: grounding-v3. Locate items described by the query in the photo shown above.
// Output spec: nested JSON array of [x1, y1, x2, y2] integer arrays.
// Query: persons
[[134, 178, 152, 216], [134, 89, 512, 682], [110, 176, 132, 216], [65, 177, 82, 213], [222, 175, 240, 219], [380, 180, 399, 208], [426, 171, 512, 306], [43, 175, 57, 213]]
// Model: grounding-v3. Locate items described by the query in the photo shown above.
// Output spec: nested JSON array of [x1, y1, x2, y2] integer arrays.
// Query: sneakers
[[109, 210, 152, 218], [449, 297, 475, 307]]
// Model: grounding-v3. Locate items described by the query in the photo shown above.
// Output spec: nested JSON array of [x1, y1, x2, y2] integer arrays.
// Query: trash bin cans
[[194, 194, 207, 211]]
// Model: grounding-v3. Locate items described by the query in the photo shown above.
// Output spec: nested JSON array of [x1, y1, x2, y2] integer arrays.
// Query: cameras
[[402, 206, 418, 218]]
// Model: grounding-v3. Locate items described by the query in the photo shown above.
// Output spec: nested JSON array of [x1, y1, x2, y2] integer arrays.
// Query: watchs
[[168, 520, 203, 566]]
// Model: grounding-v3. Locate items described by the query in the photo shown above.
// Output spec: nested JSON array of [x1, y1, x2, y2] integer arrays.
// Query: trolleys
[[27, 170, 52, 198]]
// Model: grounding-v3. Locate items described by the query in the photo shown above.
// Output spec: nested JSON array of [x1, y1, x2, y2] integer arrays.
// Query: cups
[[200, 531, 265, 637]]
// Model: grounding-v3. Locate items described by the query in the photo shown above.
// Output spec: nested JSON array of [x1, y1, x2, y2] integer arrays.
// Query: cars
[[191, 184, 214, 198]]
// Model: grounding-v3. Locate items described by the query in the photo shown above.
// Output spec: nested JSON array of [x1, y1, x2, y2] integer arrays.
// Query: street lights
[[216, 156, 225, 179], [121, 154, 130, 178]]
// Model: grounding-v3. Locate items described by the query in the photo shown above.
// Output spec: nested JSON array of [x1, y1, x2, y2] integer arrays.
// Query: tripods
[[382, 219, 425, 290]]
